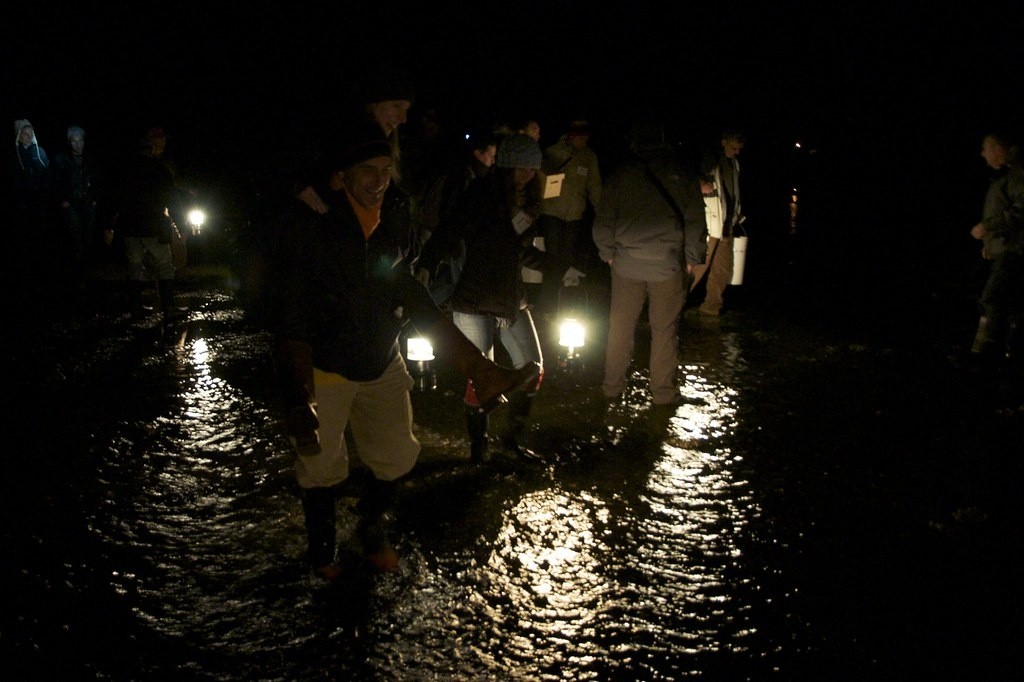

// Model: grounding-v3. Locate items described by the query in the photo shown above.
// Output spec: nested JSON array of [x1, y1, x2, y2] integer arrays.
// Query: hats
[[142, 127, 170, 146], [332, 117, 393, 172], [494, 132, 542, 170], [568, 119, 589, 136], [358, 68, 416, 105], [14, 118, 46, 171]]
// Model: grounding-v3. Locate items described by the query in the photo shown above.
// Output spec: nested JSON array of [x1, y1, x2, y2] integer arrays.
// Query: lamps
[[406, 335, 437, 393], [557, 282, 589, 372]]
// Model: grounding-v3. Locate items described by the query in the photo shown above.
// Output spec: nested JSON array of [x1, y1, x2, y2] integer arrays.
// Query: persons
[[947, 132, 1024, 374], [10, 118, 188, 299], [257, 77, 747, 566]]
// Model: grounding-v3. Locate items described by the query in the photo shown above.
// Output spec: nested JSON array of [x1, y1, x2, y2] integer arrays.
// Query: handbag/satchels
[[728, 225, 749, 287]]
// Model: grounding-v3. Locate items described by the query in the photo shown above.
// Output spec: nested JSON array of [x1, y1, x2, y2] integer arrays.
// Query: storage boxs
[[537, 167, 566, 200]]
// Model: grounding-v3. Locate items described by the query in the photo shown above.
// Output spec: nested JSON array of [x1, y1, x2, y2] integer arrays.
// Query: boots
[[128, 280, 152, 324], [465, 411, 490, 464], [157, 279, 191, 319], [503, 399, 543, 464], [300, 482, 338, 574], [423, 318, 537, 415], [356, 466, 402, 570], [282, 340, 323, 456]]
